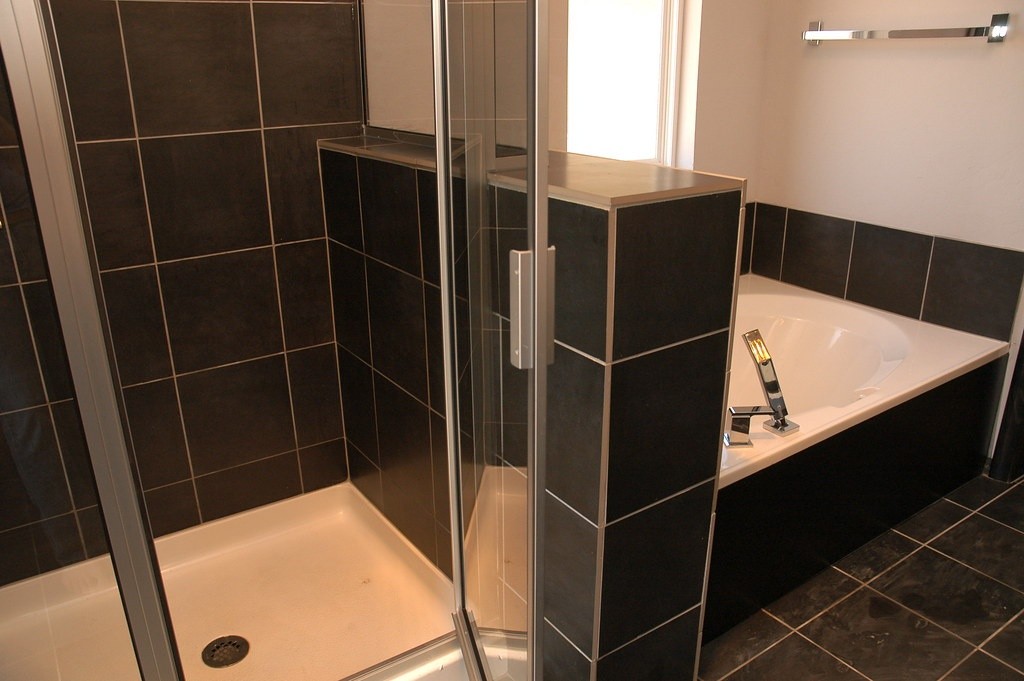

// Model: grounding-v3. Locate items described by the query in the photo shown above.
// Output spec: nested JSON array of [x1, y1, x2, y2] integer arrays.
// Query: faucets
[[723, 406, 776, 448], [741, 328, 800, 437]]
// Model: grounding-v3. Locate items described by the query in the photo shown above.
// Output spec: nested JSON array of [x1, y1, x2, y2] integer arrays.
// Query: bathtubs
[[701, 274, 1011, 647]]
